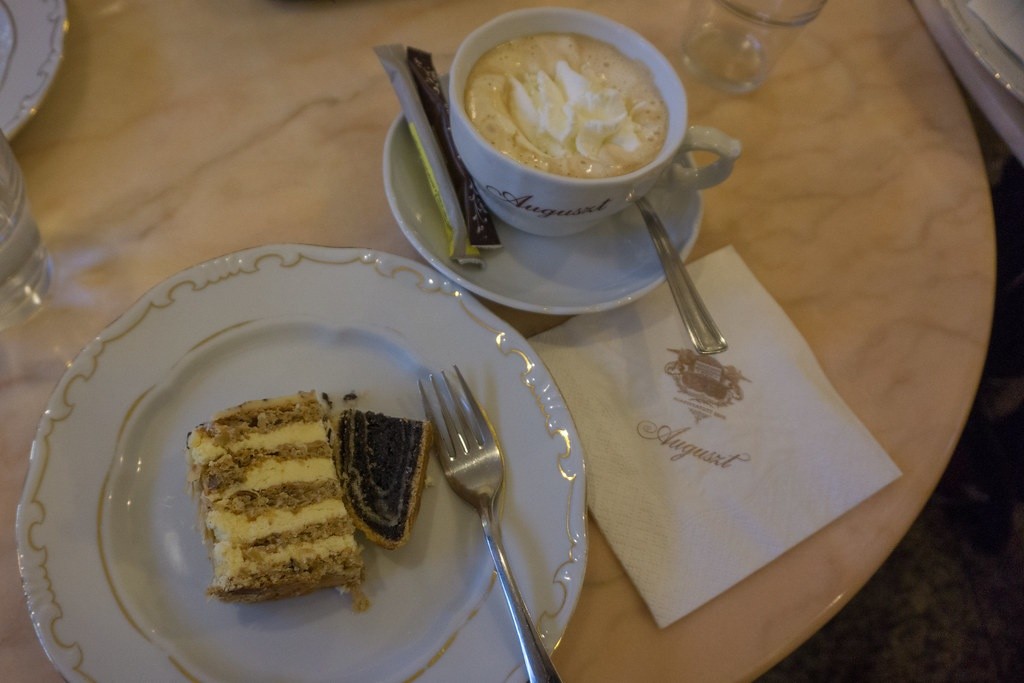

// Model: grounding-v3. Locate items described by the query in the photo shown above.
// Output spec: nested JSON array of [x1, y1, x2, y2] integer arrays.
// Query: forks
[[414, 363, 566, 682]]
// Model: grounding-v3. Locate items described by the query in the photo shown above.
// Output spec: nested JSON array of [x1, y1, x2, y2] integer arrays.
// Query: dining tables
[[0, 0, 998, 683]]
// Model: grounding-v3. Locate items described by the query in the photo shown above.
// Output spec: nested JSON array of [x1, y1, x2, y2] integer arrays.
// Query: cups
[[447, 4, 742, 240], [0, 128, 53, 326], [723, 0, 826, 24]]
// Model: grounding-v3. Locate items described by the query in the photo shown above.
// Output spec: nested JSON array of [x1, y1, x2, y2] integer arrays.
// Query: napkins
[[523, 246, 902, 627]]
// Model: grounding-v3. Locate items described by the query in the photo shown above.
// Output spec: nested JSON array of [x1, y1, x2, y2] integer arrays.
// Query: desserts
[[466, 34, 667, 178]]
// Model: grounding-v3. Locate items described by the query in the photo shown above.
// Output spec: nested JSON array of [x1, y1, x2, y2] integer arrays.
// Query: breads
[[185, 390, 430, 603]]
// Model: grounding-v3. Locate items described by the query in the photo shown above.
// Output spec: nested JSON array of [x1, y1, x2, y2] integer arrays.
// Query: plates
[[915, 0, 1024, 179], [383, 75, 704, 314], [0, 0, 67, 138], [14, 245, 587, 682]]
